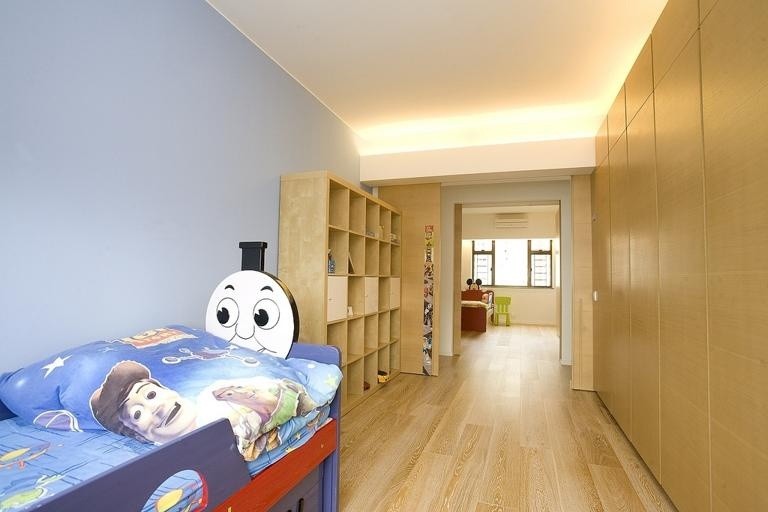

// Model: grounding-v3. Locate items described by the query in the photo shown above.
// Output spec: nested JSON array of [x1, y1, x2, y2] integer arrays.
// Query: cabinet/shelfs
[[591, 0, 767, 511], [279, 171, 403, 419]]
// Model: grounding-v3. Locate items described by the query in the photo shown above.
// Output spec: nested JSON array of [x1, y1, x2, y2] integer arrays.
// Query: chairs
[[494, 296, 512, 326]]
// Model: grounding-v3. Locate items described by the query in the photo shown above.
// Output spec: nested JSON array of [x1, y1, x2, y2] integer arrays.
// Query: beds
[[462, 279, 495, 332], [1, 240, 343, 511]]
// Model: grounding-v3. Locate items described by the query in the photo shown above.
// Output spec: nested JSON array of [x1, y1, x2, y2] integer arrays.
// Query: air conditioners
[[495, 213, 528, 228]]
